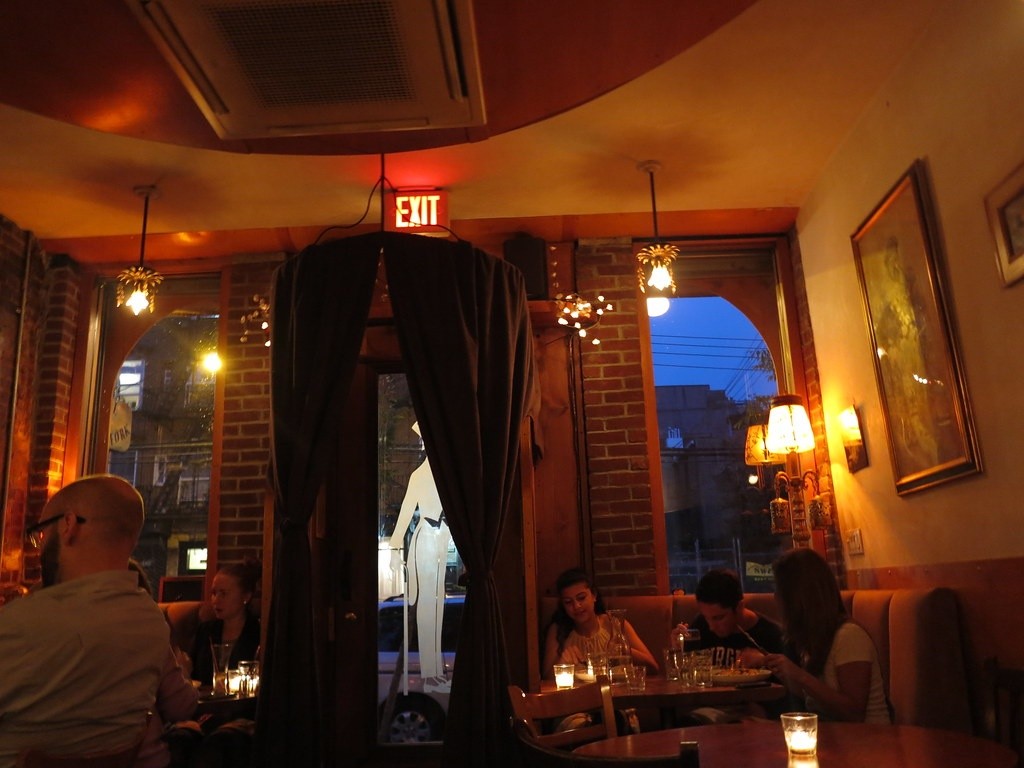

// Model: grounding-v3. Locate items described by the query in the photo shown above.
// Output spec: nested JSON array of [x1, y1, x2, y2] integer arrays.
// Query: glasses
[[26, 514, 86, 548]]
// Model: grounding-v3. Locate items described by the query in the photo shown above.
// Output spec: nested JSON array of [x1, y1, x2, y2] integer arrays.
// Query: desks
[[541, 676, 786, 732], [197, 685, 256, 713], [573, 721, 1018, 768]]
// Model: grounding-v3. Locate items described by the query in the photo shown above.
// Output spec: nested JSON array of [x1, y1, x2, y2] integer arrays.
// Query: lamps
[[115, 185, 165, 316], [635, 161, 681, 292], [765, 394, 833, 549], [739, 423, 787, 518]]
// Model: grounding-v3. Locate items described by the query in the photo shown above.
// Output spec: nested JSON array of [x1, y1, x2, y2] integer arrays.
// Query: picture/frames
[[982, 163, 1024, 288], [849, 158, 984, 496]]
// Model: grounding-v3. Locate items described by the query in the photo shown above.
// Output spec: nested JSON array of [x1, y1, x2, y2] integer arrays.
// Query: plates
[[575, 670, 607, 682], [710, 667, 773, 682]]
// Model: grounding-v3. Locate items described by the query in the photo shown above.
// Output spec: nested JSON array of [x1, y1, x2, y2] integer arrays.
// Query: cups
[[663, 647, 713, 688], [553, 653, 646, 691], [780, 712, 818, 756]]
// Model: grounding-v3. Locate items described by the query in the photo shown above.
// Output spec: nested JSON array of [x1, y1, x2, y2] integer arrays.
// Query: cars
[[378, 593, 466, 745]]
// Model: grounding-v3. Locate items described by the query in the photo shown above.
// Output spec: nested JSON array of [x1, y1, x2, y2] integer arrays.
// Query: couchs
[[543, 587, 973, 735]]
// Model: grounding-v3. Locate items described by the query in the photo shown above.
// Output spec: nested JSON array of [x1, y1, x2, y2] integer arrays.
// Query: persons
[[128, 559, 151, 596], [167, 555, 260, 768], [662, 568, 784, 731], [737, 547, 890, 724], [0, 475, 201, 768], [543, 568, 660, 749]]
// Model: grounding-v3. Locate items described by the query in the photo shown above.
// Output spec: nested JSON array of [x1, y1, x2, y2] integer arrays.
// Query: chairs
[[509, 716, 699, 768], [969, 655, 1024, 768], [506, 675, 618, 747]]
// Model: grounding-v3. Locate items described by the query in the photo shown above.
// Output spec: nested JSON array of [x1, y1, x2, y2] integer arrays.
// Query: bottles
[[210, 639, 259, 696], [606, 610, 631, 666]]
